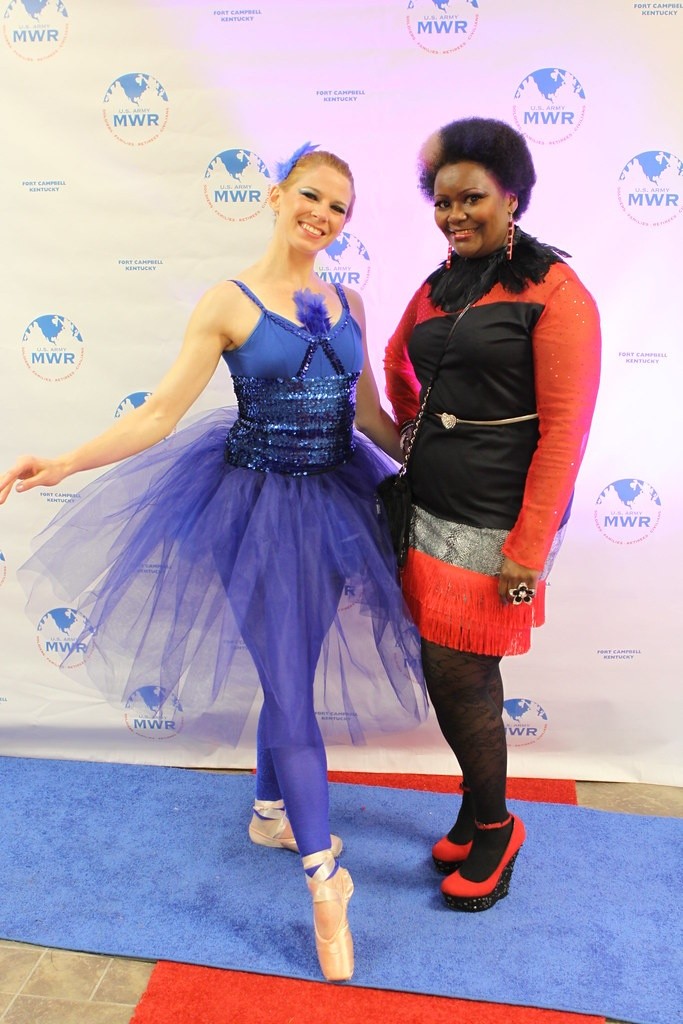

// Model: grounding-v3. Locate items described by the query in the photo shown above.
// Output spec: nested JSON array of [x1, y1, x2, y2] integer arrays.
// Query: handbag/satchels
[[379, 473, 412, 566]]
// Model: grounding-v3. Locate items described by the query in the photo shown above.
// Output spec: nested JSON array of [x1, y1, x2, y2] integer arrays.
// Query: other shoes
[[248, 799, 343, 858], [301, 848, 355, 981]]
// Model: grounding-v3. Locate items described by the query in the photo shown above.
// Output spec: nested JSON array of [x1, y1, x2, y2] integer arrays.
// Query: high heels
[[431, 809, 526, 913]]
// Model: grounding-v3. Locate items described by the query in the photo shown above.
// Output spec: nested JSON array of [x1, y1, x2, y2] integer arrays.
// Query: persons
[[375, 115, 602, 911], [1, 150, 407, 980]]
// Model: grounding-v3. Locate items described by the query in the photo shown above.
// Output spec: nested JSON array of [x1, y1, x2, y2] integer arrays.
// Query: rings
[[508, 582, 537, 605]]
